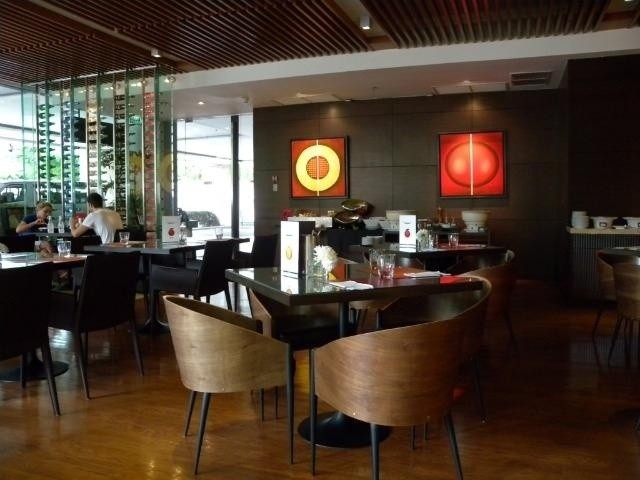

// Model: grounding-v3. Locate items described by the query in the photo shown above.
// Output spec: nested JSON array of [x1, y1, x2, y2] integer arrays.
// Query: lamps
[[359, 17, 371, 31]]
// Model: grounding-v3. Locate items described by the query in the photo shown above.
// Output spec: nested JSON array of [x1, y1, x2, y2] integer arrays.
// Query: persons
[[68, 193, 123, 244], [15, 201, 52, 234]]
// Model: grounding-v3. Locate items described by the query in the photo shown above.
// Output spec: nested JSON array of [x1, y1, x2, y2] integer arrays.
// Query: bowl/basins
[[363, 209, 417, 232], [462, 208, 491, 233], [571, 211, 589, 228], [335, 199, 368, 227], [590, 216, 640, 229]]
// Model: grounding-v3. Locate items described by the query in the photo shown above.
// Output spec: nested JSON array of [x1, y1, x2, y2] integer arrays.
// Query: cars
[[183, 209, 221, 228]]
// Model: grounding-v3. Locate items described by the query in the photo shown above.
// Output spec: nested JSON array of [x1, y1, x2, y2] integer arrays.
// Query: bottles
[[47, 215, 65, 234]]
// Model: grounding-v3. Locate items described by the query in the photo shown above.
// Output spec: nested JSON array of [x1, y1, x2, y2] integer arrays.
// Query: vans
[[0, 177, 93, 232]]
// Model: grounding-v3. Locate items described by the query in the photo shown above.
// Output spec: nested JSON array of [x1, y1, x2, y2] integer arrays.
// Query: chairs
[[608, 262, 640, 361], [592, 251, 640, 337]]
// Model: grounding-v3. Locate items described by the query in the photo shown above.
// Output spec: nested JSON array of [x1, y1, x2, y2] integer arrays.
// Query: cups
[[369, 248, 396, 280], [215, 226, 223, 240], [56, 240, 72, 258], [119, 231, 131, 244], [429, 233, 459, 249]]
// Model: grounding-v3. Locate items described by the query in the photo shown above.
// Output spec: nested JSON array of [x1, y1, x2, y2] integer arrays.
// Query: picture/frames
[[289, 137, 350, 201], [435, 128, 510, 200]]
